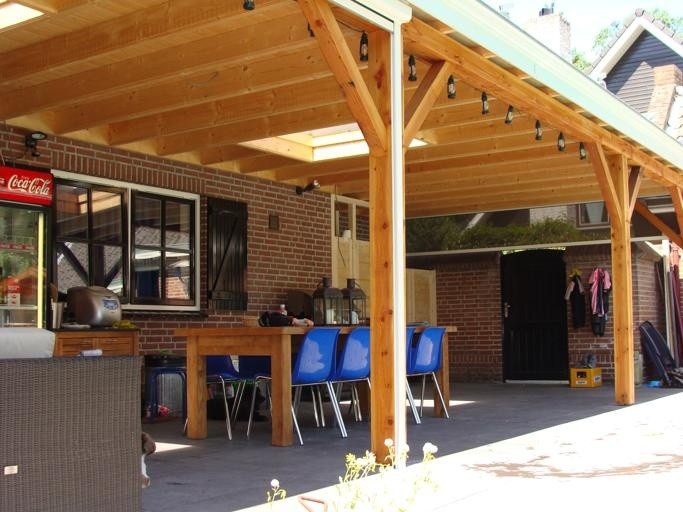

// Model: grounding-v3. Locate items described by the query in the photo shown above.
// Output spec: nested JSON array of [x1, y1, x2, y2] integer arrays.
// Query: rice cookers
[[66, 286, 121, 327]]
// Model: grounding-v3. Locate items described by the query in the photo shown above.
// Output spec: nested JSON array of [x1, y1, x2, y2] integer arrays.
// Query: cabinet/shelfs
[[45, 327, 138, 356]]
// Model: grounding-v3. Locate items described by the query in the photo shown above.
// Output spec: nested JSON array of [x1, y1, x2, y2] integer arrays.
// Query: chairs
[[200, 326, 450, 446], [0, 357, 144, 512]]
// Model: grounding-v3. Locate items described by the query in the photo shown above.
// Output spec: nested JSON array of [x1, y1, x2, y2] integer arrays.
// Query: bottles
[[279, 303, 288, 316]]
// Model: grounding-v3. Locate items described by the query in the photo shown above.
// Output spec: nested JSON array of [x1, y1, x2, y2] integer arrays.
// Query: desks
[[173, 324, 459, 447]]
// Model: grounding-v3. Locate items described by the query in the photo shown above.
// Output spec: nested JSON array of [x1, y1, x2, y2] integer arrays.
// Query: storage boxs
[[569, 366, 604, 388]]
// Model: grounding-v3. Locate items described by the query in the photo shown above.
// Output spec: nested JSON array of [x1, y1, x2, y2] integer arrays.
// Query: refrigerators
[[0, 163, 55, 328]]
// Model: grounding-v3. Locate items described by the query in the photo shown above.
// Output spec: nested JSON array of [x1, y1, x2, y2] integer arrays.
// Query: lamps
[[26, 131, 49, 148], [243, 0, 588, 161], [297, 180, 321, 195]]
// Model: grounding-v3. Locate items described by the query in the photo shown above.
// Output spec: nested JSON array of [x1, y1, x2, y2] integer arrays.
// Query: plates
[[62, 323, 90, 329]]
